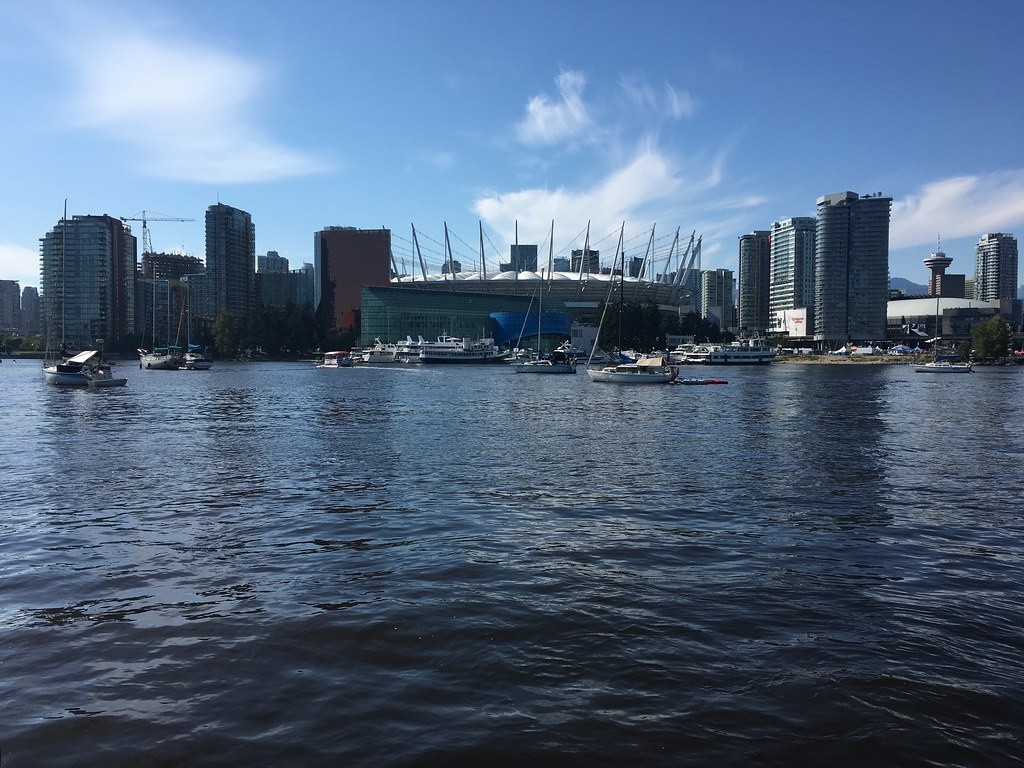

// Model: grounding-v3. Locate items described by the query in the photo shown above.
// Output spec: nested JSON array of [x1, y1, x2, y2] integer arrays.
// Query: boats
[[86, 378, 128, 388], [394, 328, 452, 363], [42, 350, 112, 385], [910, 362, 972, 373], [418, 326, 510, 364], [669, 338, 777, 365], [358, 338, 397, 363], [511, 351, 575, 373], [137, 346, 186, 369], [552, 340, 604, 363], [500, 347, 537, 364], [586, 357, 678, 383], [179, 352, 213, 370], [318, 351, 354, 368]]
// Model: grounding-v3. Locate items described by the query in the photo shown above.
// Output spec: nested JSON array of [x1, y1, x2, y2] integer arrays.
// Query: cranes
[[120, 210, 196, 273]]
